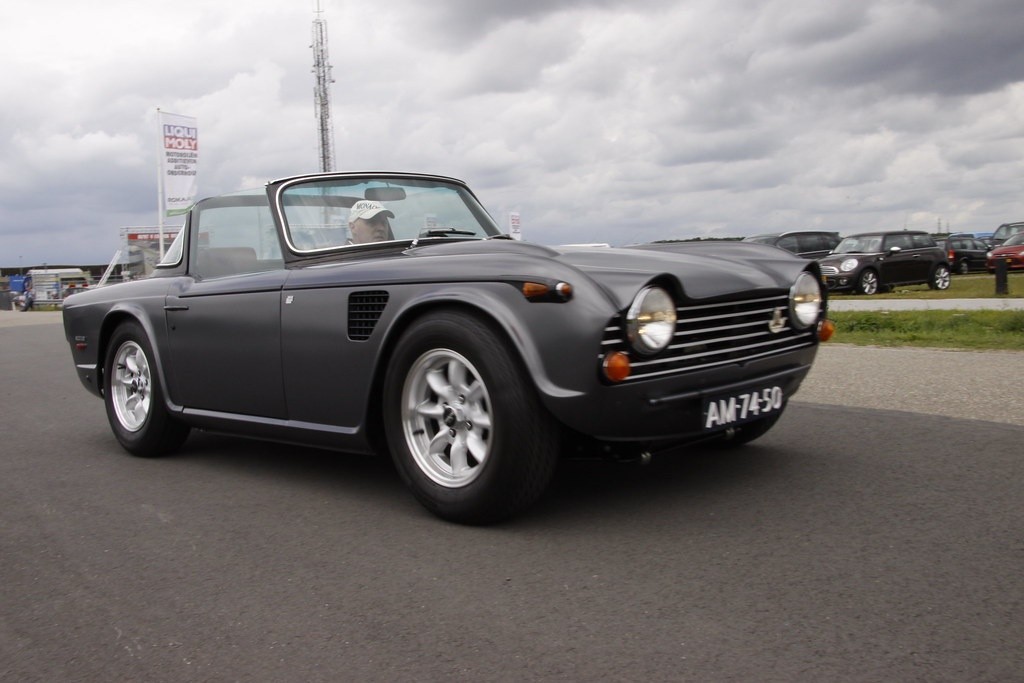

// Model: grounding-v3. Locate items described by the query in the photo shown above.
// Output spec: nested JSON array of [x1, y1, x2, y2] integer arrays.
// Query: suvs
[[819, 229, 951, 295], [739, 231, 841, 259]]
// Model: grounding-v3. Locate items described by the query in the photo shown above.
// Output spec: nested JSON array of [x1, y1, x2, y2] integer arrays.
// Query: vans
[[27, 268, 90, 300]]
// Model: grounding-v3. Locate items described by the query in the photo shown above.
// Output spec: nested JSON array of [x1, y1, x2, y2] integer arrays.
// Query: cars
[[947, 221, 1024, 241], [984, 231, 1024, 273], [934, 238, 992, 275], [61, 172, 834, 522]]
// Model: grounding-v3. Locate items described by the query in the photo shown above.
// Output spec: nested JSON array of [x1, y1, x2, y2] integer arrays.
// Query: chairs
[[194, 247, 260, 274]]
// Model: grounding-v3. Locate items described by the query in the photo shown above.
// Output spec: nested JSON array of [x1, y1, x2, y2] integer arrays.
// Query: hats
[[349, 200, 395, 222]]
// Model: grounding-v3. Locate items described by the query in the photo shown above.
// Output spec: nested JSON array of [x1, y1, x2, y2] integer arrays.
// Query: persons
[[348, 200, 394, 245]]
